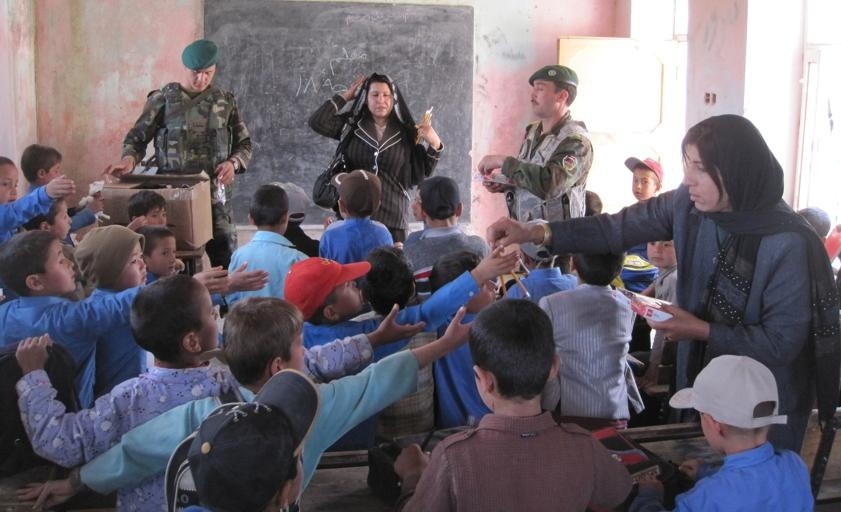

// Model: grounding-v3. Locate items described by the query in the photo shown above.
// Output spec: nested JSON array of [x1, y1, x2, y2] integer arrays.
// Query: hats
[[283, 256, 373, 323], [181, 39, 219, 70], [668, 353, 789, 431], [267, 179, 312, 224], [72, 223, 146, 290], [187, 366, 321, 512], [519, 218, 562, 261], [420, 176, 460, 222], [528, 63, 580, 88], [623, 155, 664, 185], [329, 169, 383, 217]]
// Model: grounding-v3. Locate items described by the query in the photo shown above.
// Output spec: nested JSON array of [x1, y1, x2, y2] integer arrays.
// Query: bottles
[[823, 226, 841, 263]]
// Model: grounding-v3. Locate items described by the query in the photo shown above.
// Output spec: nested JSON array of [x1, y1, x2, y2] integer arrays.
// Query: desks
[[1, 465, 63, 512], [175, 245, 207, 276]]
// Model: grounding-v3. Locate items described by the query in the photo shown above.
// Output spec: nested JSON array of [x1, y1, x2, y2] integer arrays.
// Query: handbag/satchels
[[312, 150, 352, 210], [367, 423, 471, 507]]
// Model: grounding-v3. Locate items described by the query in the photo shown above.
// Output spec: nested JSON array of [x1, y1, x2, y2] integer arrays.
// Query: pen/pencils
[[500, 273, 508, 294], [397, 428, 435, 487], [511, 269, 531, 299], [521, 260, 531, 275], [416, 106, 434, 146]]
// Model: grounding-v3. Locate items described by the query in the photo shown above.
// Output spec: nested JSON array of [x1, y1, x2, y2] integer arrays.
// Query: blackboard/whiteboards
[[204, 0, 475, 224]]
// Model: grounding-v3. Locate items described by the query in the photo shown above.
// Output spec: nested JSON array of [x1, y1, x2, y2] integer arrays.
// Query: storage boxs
[[103, 170, 214, 248]]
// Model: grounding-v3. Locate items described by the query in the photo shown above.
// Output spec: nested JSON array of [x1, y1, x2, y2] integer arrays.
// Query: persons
[[99, 39, 253, 265], [309, 73, 443, 241], [4, 114, 840, 511], [481, 65, 594, 274]]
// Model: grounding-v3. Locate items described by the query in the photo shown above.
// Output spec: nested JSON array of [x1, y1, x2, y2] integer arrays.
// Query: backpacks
[[163, 425, 303, 512], [0, 337, 83, 475], [609, 254, 661, 293], [592, 424, 695, 511]]
